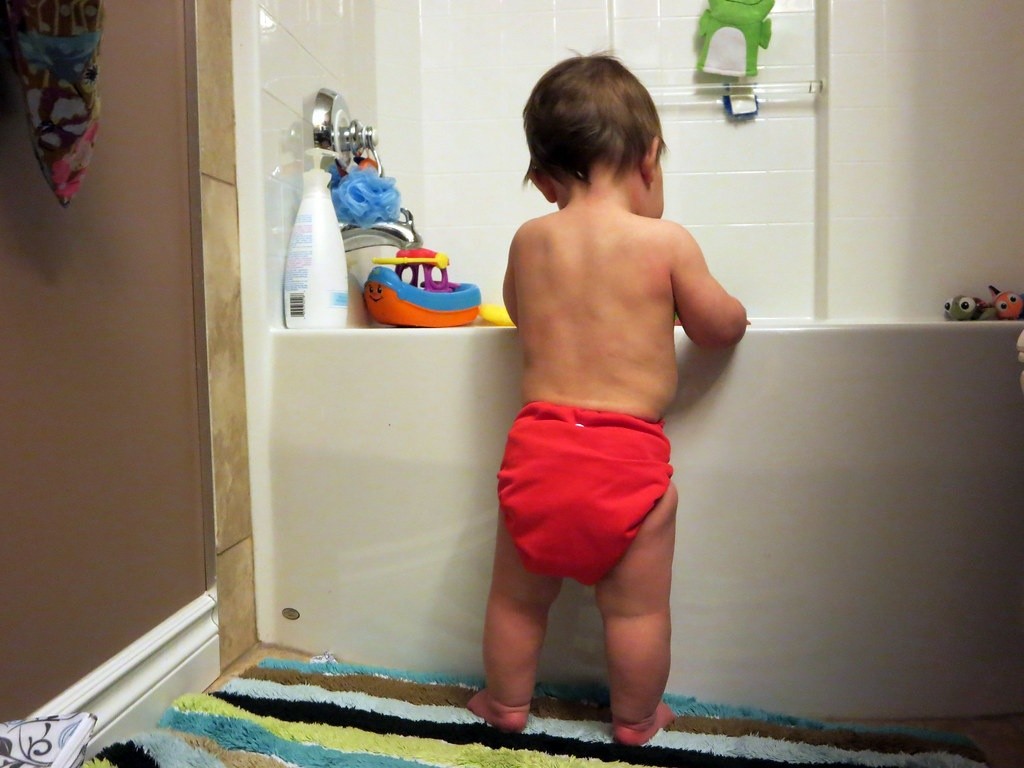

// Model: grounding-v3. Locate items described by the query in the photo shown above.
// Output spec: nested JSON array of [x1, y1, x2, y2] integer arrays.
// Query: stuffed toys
[[943, 285, 1024, 322]]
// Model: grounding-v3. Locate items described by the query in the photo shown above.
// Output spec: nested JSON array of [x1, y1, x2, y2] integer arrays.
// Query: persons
[[467, 48, 750, 746]]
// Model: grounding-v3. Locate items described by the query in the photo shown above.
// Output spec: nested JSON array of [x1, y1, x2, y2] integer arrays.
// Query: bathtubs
[[267, 319, 1024, 724]]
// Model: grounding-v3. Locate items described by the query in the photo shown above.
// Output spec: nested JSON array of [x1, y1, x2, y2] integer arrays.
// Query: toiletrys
[[281, 147, 350, 330]]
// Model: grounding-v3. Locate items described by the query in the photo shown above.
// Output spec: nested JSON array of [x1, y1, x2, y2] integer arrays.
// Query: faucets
[[339, 206, 423, 253]]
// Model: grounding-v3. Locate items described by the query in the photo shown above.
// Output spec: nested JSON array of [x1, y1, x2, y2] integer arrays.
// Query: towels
[[0, 0, 108, 210]]
